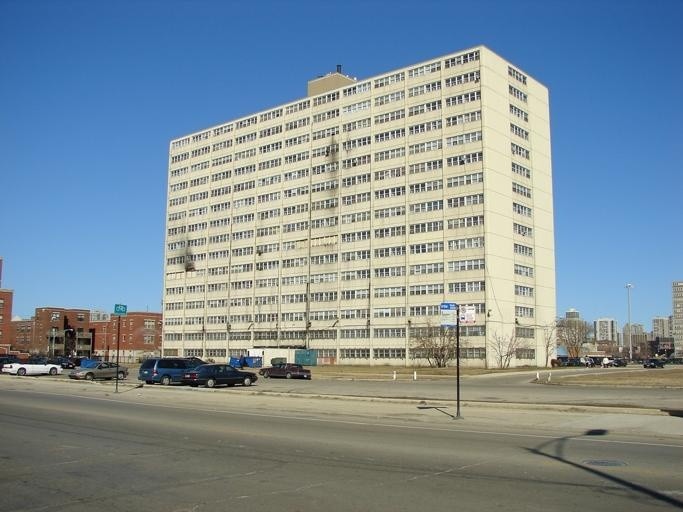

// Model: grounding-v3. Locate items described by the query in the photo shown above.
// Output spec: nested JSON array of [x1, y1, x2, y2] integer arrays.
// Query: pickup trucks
[[643, 358, 665, 369], [258, 363, 311, 380]]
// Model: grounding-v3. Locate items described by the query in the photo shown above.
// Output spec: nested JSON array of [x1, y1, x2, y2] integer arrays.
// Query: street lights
[[624, 281, 634, 363], [52, 329, 73, 357]]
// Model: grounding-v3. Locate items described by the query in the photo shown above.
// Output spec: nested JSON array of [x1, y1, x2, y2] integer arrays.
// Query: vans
[[138, 357, 208, 386]]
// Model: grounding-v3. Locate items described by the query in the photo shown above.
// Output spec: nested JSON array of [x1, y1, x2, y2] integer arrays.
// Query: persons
[[238, 354, 245, 370]]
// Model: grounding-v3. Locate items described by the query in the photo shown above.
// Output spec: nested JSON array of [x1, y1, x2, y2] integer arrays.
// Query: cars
[[561, 357, 626, 367], [182, 363, 257, 388], [67, 362, 128, 381], [0, 355, 77, 378]]
